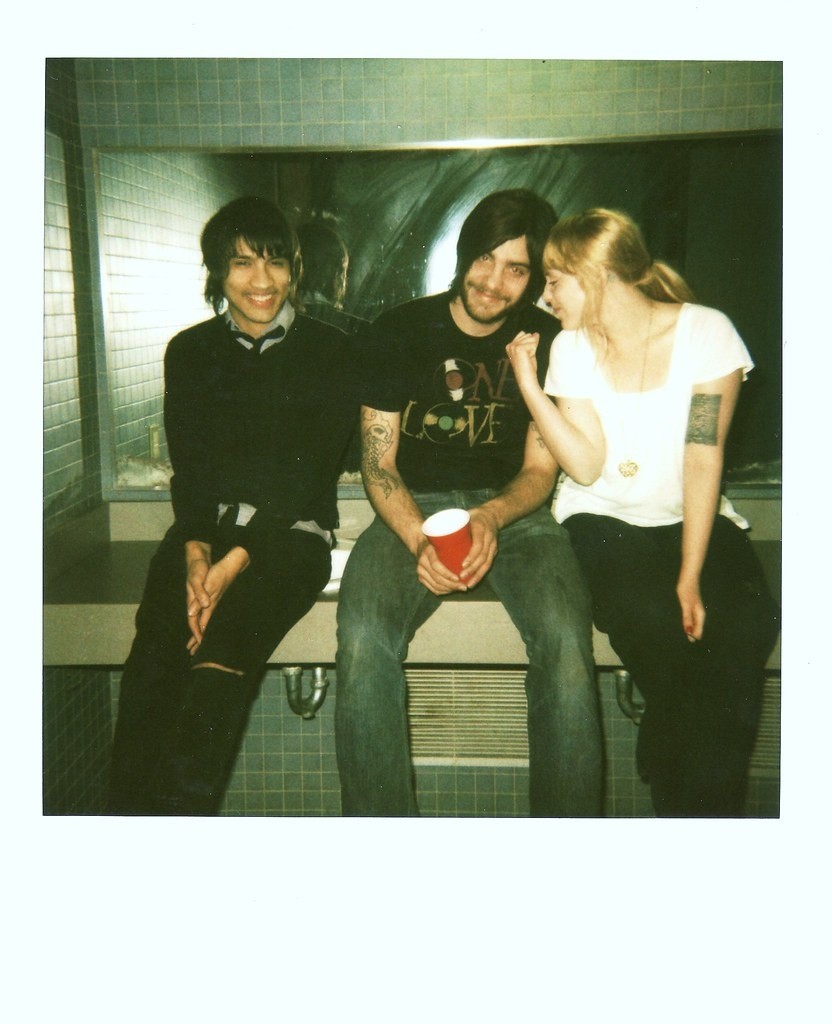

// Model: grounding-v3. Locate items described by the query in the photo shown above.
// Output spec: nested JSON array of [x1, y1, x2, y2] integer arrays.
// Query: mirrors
[[90, 126, 782, 503]]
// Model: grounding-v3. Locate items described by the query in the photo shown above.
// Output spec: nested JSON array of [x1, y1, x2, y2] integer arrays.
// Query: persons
[[334, 189, 605, 817], [506, 207, 780, 819], [107, 197, 362, 817], [291, 221, 374, 335]]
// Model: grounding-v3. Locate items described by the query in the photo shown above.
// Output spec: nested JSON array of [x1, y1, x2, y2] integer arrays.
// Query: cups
[[419, 507, 475, 587]]
